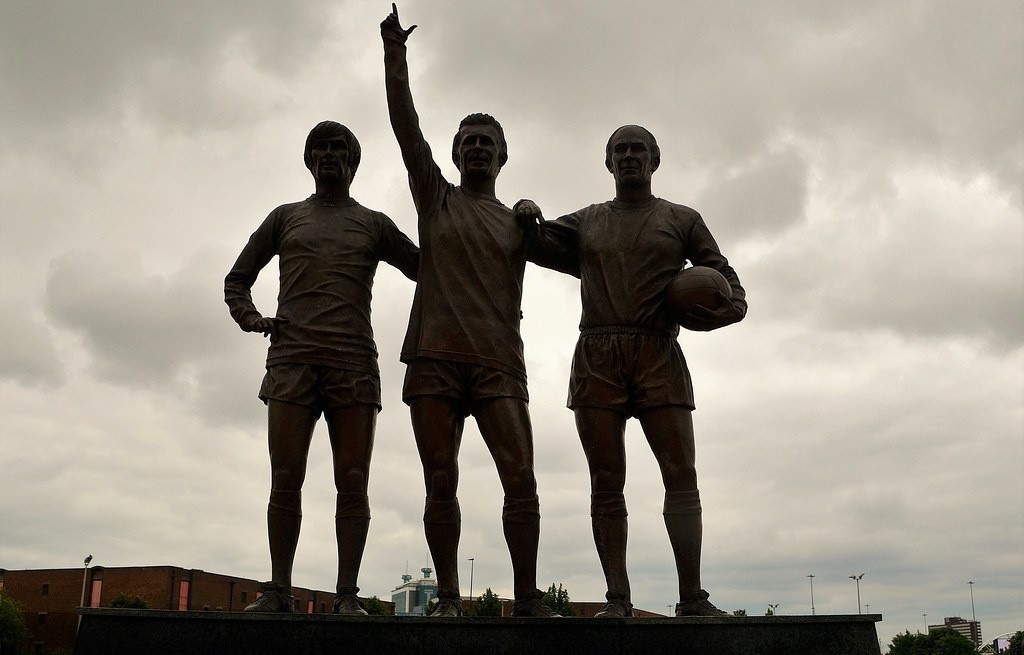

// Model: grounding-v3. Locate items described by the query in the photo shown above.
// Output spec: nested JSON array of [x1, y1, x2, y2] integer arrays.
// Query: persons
[[381, 2, 584, 617], [513, 126, 748, 619], [223, 122, 524, 615]]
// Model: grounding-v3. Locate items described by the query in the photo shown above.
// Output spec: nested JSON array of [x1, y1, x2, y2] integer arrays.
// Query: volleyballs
[[666, 265, 733, 332]]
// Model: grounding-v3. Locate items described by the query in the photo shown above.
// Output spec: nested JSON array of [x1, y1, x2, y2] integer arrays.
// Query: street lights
[[967, 581, 979, 651], [76, 555, 93, 636], [807, 574, 815, 615], [768, 604, 778, 615], [849, 573, 865, 614], [468, 558, 474, 612]]
